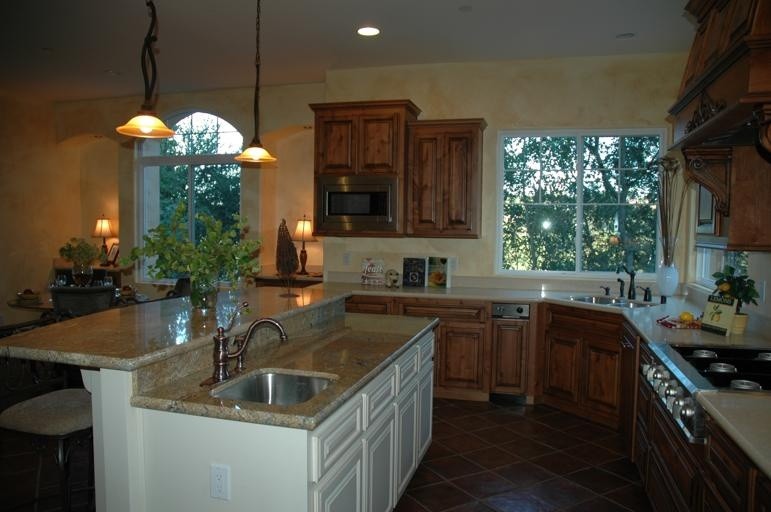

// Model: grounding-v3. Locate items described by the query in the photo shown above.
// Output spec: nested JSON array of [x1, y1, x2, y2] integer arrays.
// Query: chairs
[[49, 288, 113, 321]]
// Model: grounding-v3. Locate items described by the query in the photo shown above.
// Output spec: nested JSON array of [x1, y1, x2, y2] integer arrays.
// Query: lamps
[[230, 0, 280, 164], [91, 213, 113, 266], [291, 214, 319, 274], [116, 0, 172, 140]]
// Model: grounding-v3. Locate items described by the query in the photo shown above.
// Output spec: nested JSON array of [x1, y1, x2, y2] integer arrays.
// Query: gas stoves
[[640, 338, 771, 447]]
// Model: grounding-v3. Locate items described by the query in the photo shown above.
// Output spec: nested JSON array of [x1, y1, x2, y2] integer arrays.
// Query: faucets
[[616, 265, 637, 299], [213, 302, 289, 382]]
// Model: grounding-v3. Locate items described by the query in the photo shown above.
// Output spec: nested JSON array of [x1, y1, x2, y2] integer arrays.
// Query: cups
[[103, 276, 112, 286], [115, 288, 121, 298], [93, 280, 103, 288], [57, 274, 66, 287]]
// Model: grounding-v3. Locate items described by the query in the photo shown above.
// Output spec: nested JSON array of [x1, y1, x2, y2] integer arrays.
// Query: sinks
[[210, 367, 342, 406], [565, 296, 658, 310]]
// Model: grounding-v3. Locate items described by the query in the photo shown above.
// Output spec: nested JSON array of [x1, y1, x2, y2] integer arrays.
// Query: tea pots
[[123, 284, 137, 295]]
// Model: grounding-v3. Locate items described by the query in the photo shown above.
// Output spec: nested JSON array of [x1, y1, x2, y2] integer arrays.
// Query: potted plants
[[712, 266, 760, 336], [643, 153, 689, 297], [116, 200, 263, 310], [57, 236, 99, 286]]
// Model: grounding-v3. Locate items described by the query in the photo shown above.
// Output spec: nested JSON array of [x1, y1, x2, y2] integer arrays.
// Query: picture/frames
[[106, 243, 119, 264]]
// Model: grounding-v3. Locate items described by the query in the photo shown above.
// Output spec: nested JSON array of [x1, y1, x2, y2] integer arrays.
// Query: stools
[[0, 388, 94, 511]]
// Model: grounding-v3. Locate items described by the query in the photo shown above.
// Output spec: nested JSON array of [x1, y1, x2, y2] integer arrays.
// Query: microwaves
[[315, 172, 398, 234]]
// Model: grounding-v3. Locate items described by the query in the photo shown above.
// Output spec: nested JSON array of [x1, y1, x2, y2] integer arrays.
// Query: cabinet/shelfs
[[544, 304, 624, 416], [632, 373, 695, 512], [345, 294, 394, 314], [310, 365, 394, 512], [694, 410, 771, 512], [407, 125, 484, 239], [492, 319, 527, 394], [314, 106, 417, 176], [394, 329, 435, 508], [619, 322, 640, 464], [393, 297, 492, 402]]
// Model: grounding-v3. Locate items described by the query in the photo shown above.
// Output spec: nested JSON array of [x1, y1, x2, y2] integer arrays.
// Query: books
[[361, 254, 452, 289], [700, 294, 738, 336]]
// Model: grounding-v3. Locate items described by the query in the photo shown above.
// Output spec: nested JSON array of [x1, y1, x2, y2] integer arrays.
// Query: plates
[[16, 291, 40, 300]]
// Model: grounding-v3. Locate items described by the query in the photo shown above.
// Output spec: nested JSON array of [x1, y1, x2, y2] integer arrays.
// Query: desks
[[0, 306, 43, 339], [7, 291, 149, 314], [256, 272, 323, 287], [53, 263, 135, 289]]
